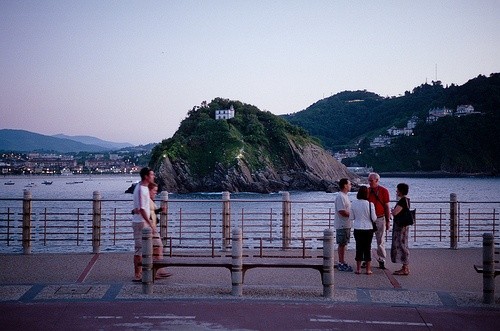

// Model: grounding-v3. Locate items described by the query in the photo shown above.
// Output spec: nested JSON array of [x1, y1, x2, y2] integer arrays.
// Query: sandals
[[394, 269, 410, 275]]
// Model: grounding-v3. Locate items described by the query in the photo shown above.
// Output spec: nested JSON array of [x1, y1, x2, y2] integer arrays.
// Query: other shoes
[[379, 261, 386, 270], [158, 272, 172, 277], [337, 263, 353, 272], [133, 279, 143, 283], [361, 262, 366, 268]]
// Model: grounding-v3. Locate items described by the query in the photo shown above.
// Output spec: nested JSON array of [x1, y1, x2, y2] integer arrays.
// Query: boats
[[41, 179, 53, 185], [4, 179, 15, 185]]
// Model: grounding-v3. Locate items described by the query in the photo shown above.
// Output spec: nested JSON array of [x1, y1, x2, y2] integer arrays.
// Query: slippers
[[354, 272, 362, 274], [366, 271, 373, 274]]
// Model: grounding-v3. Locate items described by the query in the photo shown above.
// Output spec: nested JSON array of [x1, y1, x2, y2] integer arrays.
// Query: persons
[[350, 186, 377, 274], [334, 178, 354, 272], [360, 173, 390, 270], [131, 167, 171, 282], [390, 182, 411, 276]]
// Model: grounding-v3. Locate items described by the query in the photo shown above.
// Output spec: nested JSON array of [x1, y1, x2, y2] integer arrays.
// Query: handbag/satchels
[[395, 197, 414, 227], [369, 202, 378, 232]]
